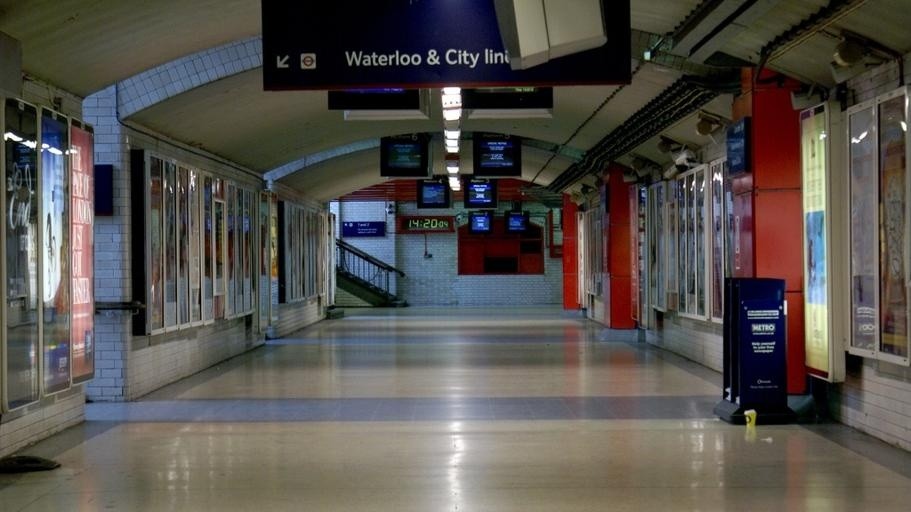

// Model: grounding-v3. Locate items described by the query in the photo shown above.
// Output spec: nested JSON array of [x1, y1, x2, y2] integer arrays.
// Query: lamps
[[441, 86, 464, 192], [627, 151, 662, 170], [657, 134, 679, 153], [695, 114, 721, 136]]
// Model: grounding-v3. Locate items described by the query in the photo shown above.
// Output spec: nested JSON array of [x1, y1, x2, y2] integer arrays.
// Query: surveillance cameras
[[412, 133, 418, 140], [505, 131, 510, 140], [385, 208, 393, 215], [456, 214, 462, 223]]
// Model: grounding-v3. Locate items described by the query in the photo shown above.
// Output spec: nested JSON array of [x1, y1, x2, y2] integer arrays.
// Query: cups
[[745, 427, 756, 443], [744, 409, 757, 427]]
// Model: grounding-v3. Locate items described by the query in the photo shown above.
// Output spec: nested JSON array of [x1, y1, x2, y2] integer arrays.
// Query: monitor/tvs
[[416, 176, 450, 209], [464, 177, 498, 208], [327, 87, 421, 110], [461, 87, 554, 110], [468, 211, 496, 235], [504, 210, 530, 234], [380, 132, 430, 178], [472, 130, 522, 177]]
